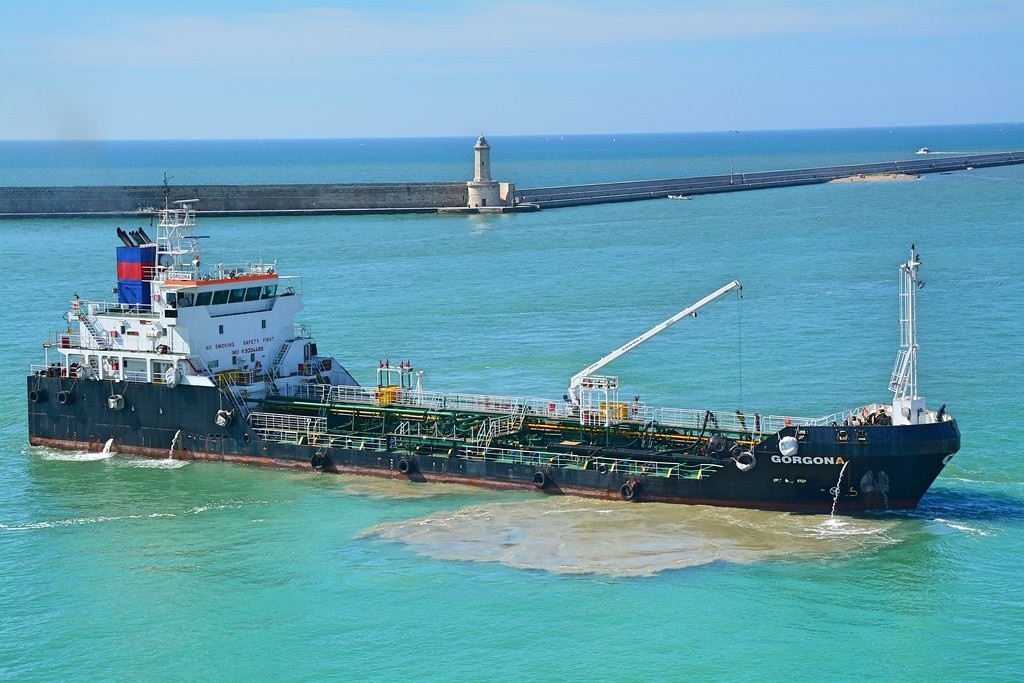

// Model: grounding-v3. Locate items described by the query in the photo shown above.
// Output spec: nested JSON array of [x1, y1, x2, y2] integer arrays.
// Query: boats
[[25, 170, 962, 514]]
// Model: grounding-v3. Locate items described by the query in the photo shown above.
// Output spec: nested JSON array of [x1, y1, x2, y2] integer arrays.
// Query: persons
[[937, 404, 946, 423]]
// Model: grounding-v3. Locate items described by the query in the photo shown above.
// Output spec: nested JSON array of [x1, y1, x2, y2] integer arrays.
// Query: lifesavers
[[310, 453, 325, 470], [397, 459, 410, 475], [619, 483, 635, 501], [598, 464, 608, 475], [862, 407, 868, 418], [533, 472, 546, 489], [29, 390, 40, 403], [57, 391, 69, 405]]
